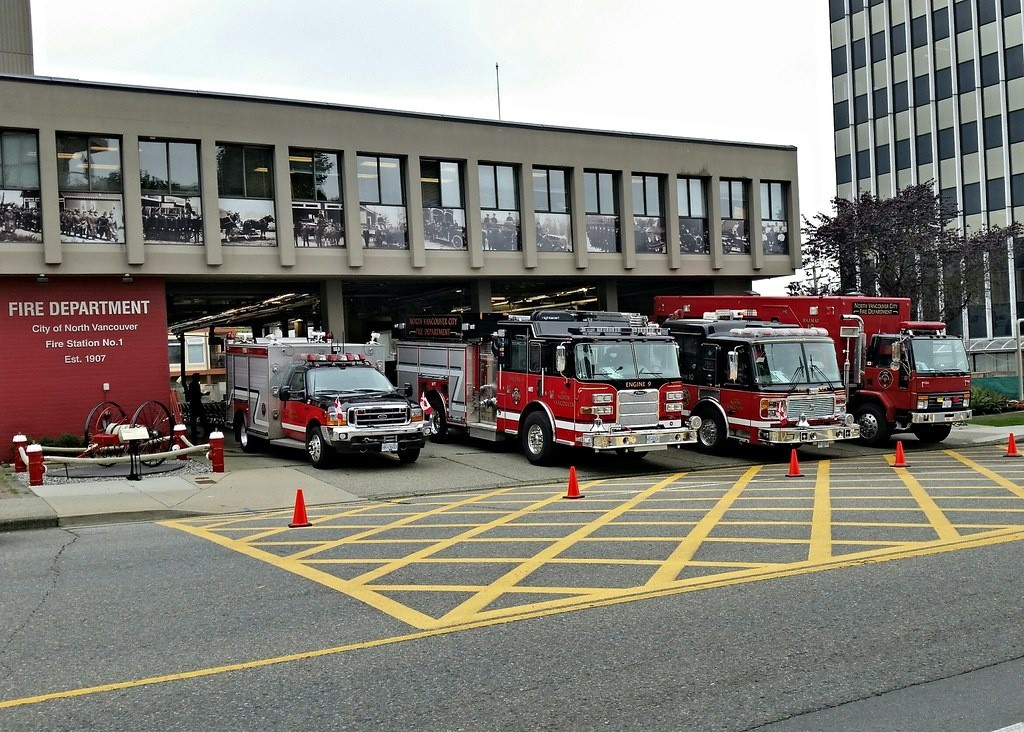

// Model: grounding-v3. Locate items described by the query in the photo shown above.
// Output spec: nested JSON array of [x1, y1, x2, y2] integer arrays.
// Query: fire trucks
[[653, 294, 973, 449], [655, 307, 861, 461], [224, 330, 431, 469]]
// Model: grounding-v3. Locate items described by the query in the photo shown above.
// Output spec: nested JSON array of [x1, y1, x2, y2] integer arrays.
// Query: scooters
[[393, 304, 702, 468]]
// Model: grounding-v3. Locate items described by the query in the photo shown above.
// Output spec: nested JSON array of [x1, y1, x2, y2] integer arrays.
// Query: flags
[[775, 402, 788, 426], [334, 394, 343, 421], [419, 391, 434, 415]]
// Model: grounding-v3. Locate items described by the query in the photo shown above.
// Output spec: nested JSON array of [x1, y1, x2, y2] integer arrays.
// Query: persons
[[187, 373, 211, 445], [363, 214, 408, 247], [731, 221, 740, 239], [587, 217, 620, 252], [295, 211, 342, 247], [681, 224, 709, 254], [665, 314, 673, 321], [634, 223, 666, 254], [424, 217, 458, 239], [0, 204, 42, 233], [536, 220, 572, 252], [481, 211, 518, 249], [491, 331, 501, 359], [763, 221, 787, 254], [59, 208, 118, 242], [144, 200, 202, 243]]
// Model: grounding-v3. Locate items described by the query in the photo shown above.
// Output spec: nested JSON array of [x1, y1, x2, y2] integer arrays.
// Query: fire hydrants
[[174, 424, 189, 459], [12, 435, 28, 472], [207, 431, 225, 473], [27, 444, 46, 486]]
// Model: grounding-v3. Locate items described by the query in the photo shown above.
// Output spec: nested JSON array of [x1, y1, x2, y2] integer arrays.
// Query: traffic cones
[[888, 440, 912, 467], [560, 466, 586, 499], [285, 491, 313, 530], [783, 447, 806, 477], [1002, 432, 1024, 458]]
